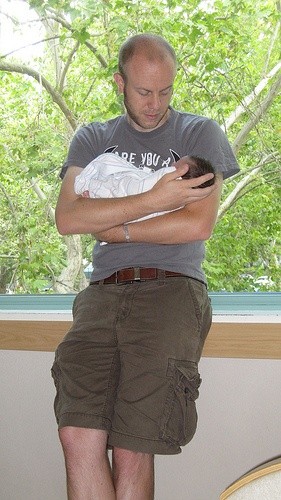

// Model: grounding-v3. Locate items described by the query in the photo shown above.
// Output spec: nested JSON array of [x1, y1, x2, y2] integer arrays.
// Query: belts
[[90, 267, 180, 286]]
